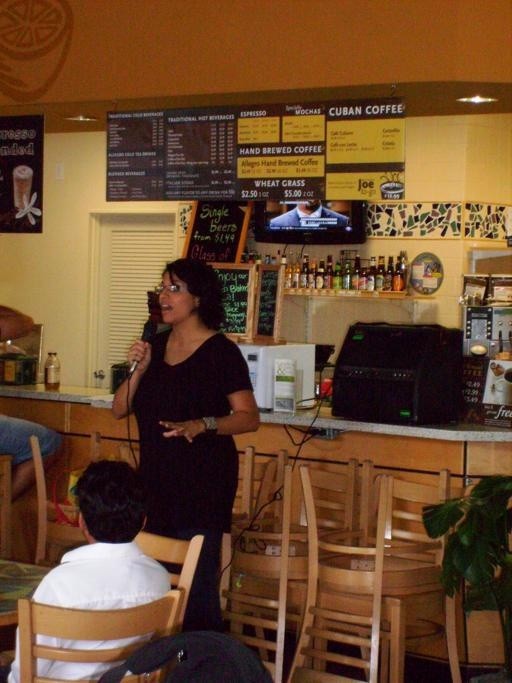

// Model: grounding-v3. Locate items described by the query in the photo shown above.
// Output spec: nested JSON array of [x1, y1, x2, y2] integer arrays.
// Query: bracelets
[[202, 416, 218, 436]]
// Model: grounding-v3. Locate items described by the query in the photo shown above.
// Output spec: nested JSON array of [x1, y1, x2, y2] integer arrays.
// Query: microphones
[[124, 322, 154, 372]]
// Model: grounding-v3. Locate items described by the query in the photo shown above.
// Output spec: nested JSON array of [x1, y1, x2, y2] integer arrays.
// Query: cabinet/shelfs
[[282, 286, 407, 380]]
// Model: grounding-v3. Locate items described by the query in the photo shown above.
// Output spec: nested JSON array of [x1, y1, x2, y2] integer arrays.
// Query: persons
[[0, 304, 64, 504], [111, 259, 261, 632], [270, 200, 349, 229], [8, 460, 171, 683]]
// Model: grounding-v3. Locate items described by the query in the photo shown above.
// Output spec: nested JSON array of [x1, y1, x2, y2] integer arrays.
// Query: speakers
[[331, 323, 463, 426]]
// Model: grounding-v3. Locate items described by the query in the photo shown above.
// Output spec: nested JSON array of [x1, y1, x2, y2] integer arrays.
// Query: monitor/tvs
[[250, 196, 368, 241]]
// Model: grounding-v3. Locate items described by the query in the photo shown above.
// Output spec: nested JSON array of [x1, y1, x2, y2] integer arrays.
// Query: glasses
[[156, 282, 188, 295]]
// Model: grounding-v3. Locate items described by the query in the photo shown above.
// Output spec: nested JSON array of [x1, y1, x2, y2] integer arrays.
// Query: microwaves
[[237, 343, 314, 409]]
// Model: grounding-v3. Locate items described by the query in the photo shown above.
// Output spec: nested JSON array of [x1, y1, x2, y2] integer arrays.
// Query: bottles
[[375, 255, 386, 292], [240, 252, 247, 265], [276, 249, 282, 266], [282, 254, 287, 264], [265, 255, 271, 265], [285, 263, 293, 290], [342, 259, 353, 290], [44, 351, 61, 393], [332, 259, 342, 290], [247, 252, 255, 265], [292, 262, 300, 289], [368, 257, 378, 291], [271, 256, 277, 265], [384, 256, 395, 292], [307, 262, 317, 289], [393, 256, 406, 291], [325, 255, 333, 289], [316, 258, 325, 290], [360, 264, 368, 290], [299, 253, 311, 289], [352, 254, 361, 290]]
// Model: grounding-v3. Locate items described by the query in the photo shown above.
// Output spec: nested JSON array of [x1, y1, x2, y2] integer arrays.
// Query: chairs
[[288, 468, 467, 682], [220, 445, 356, 682], [19, 590, 185, 683], [29, 431, 100, 566], [133, 531, 204, 592]]
